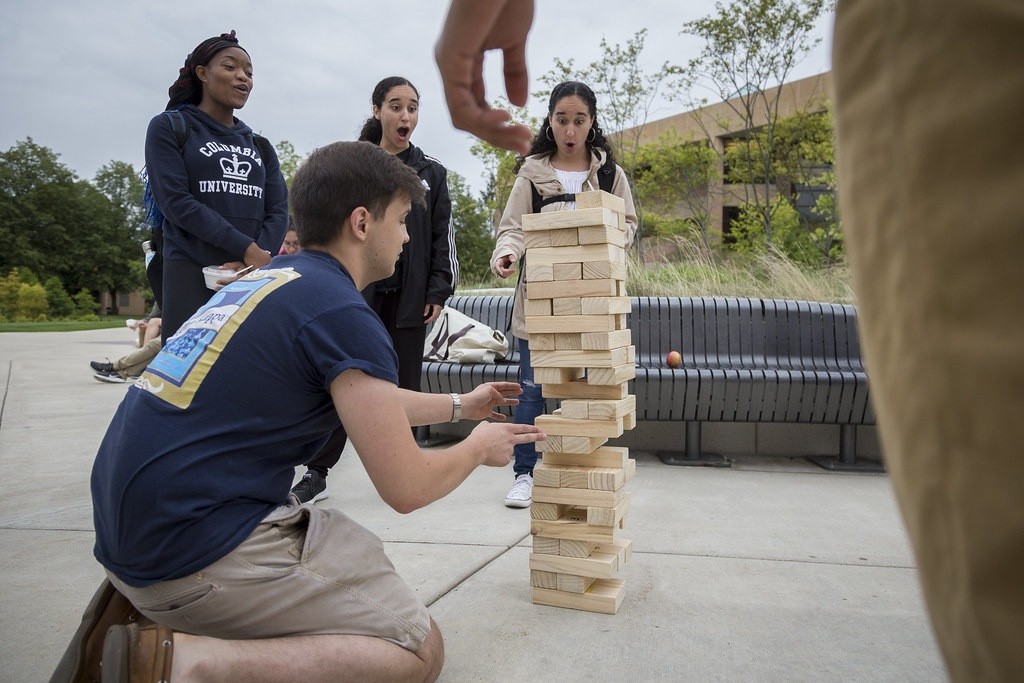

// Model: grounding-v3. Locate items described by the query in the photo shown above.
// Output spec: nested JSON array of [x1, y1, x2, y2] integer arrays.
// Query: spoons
[[221, 251, 271, 278]]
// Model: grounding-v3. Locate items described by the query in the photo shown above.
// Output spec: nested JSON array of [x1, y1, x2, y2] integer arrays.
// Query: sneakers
[[93, 369, 126, 383], [101, 621, 173, 683], [49, 576, 141, 683], [504, 472, 534, 507], [289, 469, 327, 505], [90, 357, 113, 371]]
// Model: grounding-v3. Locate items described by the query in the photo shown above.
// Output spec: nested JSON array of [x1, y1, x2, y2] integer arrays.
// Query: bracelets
[[449, 393, 461, 424]]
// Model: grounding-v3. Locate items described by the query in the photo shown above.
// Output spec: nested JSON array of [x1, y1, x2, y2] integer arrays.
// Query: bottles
[[142, 241, 155, 270]]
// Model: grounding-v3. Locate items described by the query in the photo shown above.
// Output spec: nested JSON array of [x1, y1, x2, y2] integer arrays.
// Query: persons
[[90, 300, 162, 382], [144, 29, 287, 343], [489, 80, 638, 508], [284, 224, 301, 255], [71, 141, 547, 683], [433, 0, 1024, 683], [291, 76, 459, 503]]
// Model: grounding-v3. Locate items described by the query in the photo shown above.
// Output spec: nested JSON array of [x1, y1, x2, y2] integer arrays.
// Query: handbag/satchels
[[423, 304, 510, 365]]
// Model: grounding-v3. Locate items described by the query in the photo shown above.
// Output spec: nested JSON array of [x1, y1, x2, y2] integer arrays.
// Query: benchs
[[417, 296, 885, 472]]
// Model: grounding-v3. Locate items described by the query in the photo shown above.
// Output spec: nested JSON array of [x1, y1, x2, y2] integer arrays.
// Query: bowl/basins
[[125, 319, 136, 327], [202, 265, 236, 290]]
